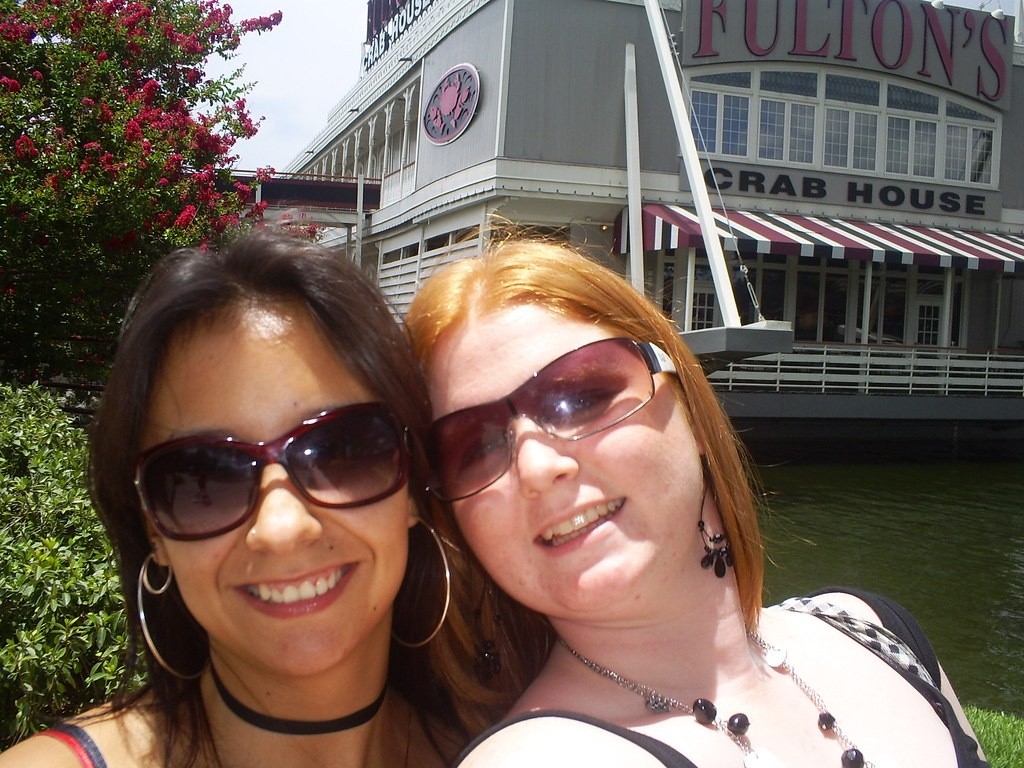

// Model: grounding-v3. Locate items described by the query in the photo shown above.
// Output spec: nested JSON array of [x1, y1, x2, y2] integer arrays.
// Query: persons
[[0, 223, 470, 768], [401, 209, 991, 768]]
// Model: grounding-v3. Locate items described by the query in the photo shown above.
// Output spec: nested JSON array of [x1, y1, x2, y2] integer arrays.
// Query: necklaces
[[553, 630, 874, 768], [207, 647, 390, 735]]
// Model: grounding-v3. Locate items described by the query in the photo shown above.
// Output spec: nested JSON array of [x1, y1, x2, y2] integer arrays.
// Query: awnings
[[611, 202, 1024, 279]]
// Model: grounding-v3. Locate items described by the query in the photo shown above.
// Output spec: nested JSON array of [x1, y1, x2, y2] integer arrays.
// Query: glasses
[[133, 401, 414, 541], [421, 338, 676, 503]]
